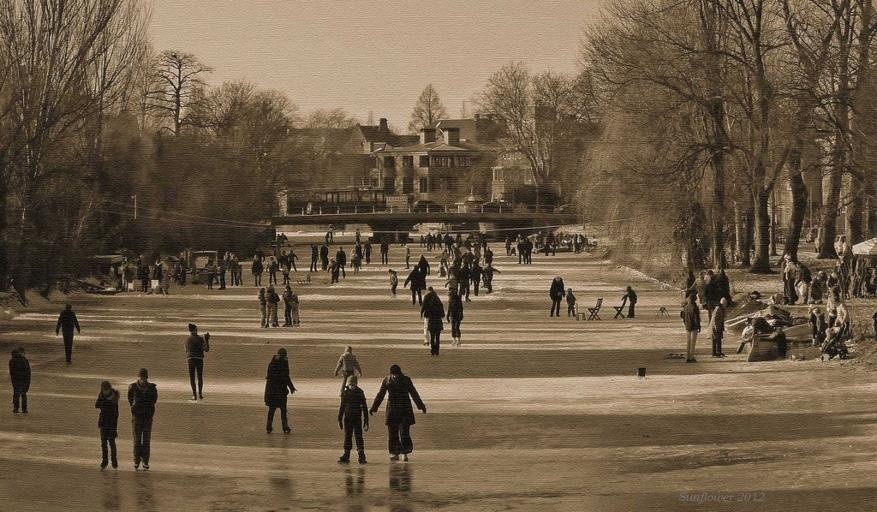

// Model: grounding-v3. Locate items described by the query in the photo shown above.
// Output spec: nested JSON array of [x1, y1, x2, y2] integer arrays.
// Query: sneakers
[[359, 455, 366, 461], [391, 452, 407, 460], [191, 396, 197, 400], [99, 460, 152, 468], [282, 426, 291, 431], [686, 358, 696, 362], [340, 452, 350, 460], [199, 395, 203, 398], [266, 426, 272, 432]]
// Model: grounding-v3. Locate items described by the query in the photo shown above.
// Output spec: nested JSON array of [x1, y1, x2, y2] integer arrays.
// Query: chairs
[[575, 303, 585, 320], [587, 298, 604, 321], [613, 297, 627, 319]]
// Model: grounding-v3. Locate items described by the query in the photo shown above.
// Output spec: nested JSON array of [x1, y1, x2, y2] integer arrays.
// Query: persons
[[121, 222, 372, 328], [56, 305, 80, 365], [9, 348, 31, 413], [264, 348, 296, 433], [622, 287, 637, 318], [503, 229, 588, 266], [95, 382, 119, 470], [566, 288, 576, 316], [681, 235, 877, 363], [184, 323, 209, 402], [402, 233, 493, 358], [127, 368, 158, 472], [389, 269, 398, 298], [337, 375, 370, 464], [550, 277, 566, 316], [334, 346, 362, 397], [369, 365, 427, 461], [380, 241, 388, 266]]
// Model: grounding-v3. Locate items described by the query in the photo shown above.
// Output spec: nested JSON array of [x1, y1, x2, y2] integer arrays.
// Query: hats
[[720, 298, 727, 304], [278, 348, 287, 356], [390, 365, 401, 374], [688, 294, 697, 300], [347, 375, 357, 385], [344, 346, 352, 353], [188, 323, 197, 332], [137, 368, 148, 378]]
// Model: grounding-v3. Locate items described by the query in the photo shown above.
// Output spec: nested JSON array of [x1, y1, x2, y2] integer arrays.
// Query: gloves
[[422, 407, 426, 413], [369, 409, 376, 415], [337, 422, 343, 429], [204, 332, 210, 341], [363, 424, 369, 432]]
